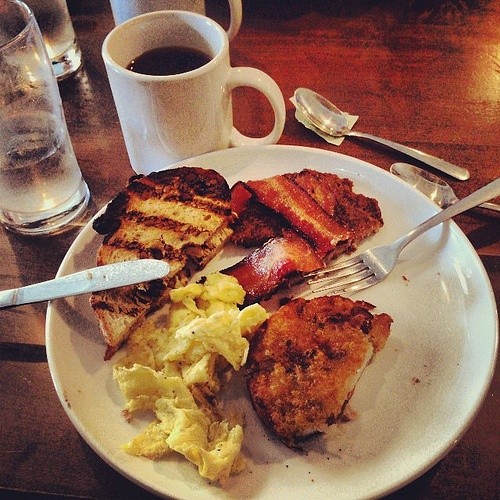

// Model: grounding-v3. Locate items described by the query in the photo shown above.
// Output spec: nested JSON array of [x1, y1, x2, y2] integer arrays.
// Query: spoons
[[294, 86, 471, 181], [390, 161, 500, 212]]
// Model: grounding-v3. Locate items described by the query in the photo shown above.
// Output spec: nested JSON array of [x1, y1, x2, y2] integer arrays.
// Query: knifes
[[0, 258, 171, 309]]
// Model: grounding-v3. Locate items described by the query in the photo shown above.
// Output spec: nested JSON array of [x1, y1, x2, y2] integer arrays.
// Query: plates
[[43, 143, 500, 500]]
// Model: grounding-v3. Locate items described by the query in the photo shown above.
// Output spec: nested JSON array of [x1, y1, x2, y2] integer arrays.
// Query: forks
[[302, 175, 500, 298]]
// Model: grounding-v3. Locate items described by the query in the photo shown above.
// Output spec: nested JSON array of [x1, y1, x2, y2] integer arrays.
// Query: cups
[[101, 9, 287, 177], [109, 0, 242, 43], [0, 0, 91, 238], [22, 0, 83, 83]]
